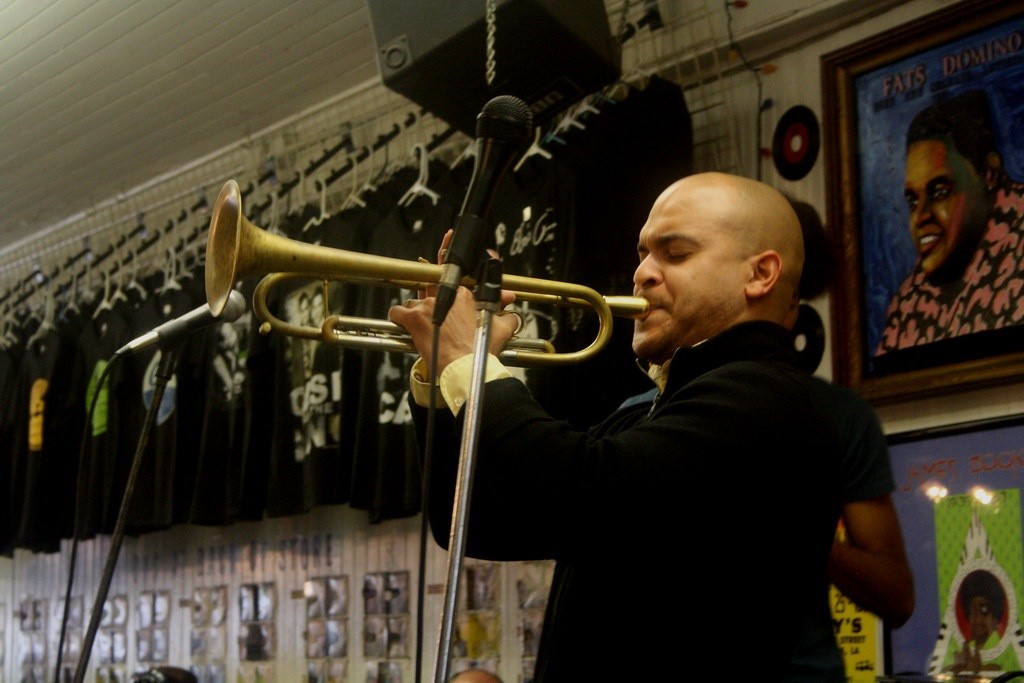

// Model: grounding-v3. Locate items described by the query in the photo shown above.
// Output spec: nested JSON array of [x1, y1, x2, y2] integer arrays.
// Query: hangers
[[0, 14, 652, 351]]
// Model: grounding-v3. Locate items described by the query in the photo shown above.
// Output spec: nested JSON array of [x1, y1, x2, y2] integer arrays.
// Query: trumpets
[[203, 180, 648, 368]]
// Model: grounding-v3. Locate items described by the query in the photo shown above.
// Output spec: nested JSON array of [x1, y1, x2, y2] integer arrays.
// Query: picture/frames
[[819, 0, 1024, 407], [883, 411, 1024, 683]]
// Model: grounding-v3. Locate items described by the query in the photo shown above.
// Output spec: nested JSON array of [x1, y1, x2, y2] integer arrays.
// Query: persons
[[962, 571, 1004, 648], [391, 172, 915, 683], [877, 90, 1024, 355], [212, 286, 340, 445]]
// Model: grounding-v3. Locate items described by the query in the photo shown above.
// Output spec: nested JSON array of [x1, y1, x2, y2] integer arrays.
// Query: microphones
[[432, 92, 534, 327], [114, 288, 247, 357]]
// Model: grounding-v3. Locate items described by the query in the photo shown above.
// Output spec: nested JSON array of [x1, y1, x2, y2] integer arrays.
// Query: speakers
[[365, 0, 620, 141]]
